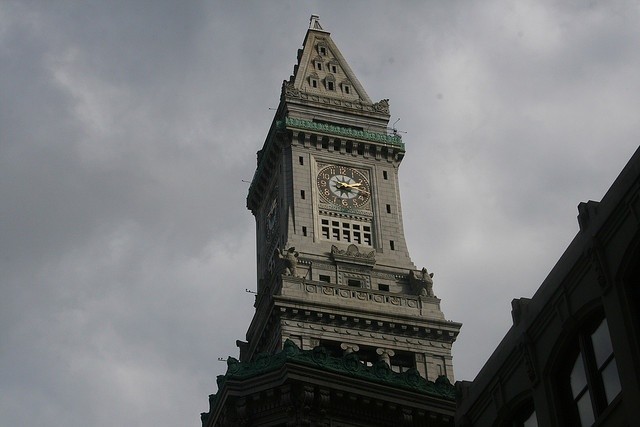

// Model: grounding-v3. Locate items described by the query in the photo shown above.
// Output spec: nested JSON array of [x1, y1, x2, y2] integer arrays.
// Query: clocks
[[315, 162, 372, 209], [264, 192, 281, 241]]
